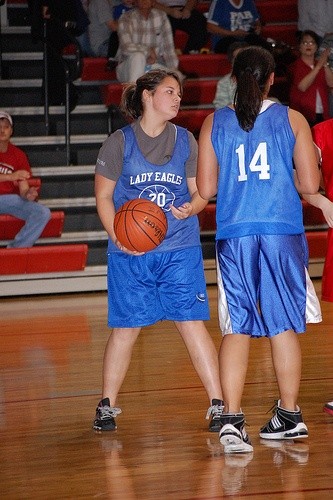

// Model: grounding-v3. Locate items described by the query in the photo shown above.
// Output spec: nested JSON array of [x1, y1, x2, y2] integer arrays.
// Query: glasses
[[303, 41, 314, 46]]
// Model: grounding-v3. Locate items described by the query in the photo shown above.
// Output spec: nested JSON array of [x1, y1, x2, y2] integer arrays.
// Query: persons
[[284, 30, 333, 128], [212, 41, 258, 109], [301, 112, 333, 416], [0, 110, 52, 250], [195, 48, 319, 453], [93, 68, 240, 432], [30, 0, 275, 85], [296, 0, 333, 38]]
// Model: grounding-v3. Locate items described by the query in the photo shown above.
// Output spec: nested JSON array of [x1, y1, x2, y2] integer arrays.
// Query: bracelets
[[324, 63, 329, 67]]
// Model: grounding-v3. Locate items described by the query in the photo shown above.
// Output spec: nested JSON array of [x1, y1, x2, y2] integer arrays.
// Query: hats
[[0, 111, 13, 125]]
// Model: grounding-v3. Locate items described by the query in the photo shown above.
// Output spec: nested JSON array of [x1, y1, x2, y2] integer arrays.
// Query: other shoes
[[106, 60, 117, 71]]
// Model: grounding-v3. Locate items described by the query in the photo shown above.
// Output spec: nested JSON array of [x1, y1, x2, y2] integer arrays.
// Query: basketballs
[[113, 199, 168, 252]]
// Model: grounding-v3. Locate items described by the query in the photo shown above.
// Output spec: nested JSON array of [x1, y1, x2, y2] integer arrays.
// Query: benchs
[[0, 0, 328, 296]]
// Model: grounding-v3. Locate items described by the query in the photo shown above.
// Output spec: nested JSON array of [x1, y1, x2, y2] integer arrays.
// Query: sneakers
[[323, 401, 333, 415], [205, 398, 224, 432], [218, 414, 254, 452], [93, 398, 121, 431], [259, 399, 308, 439]]
